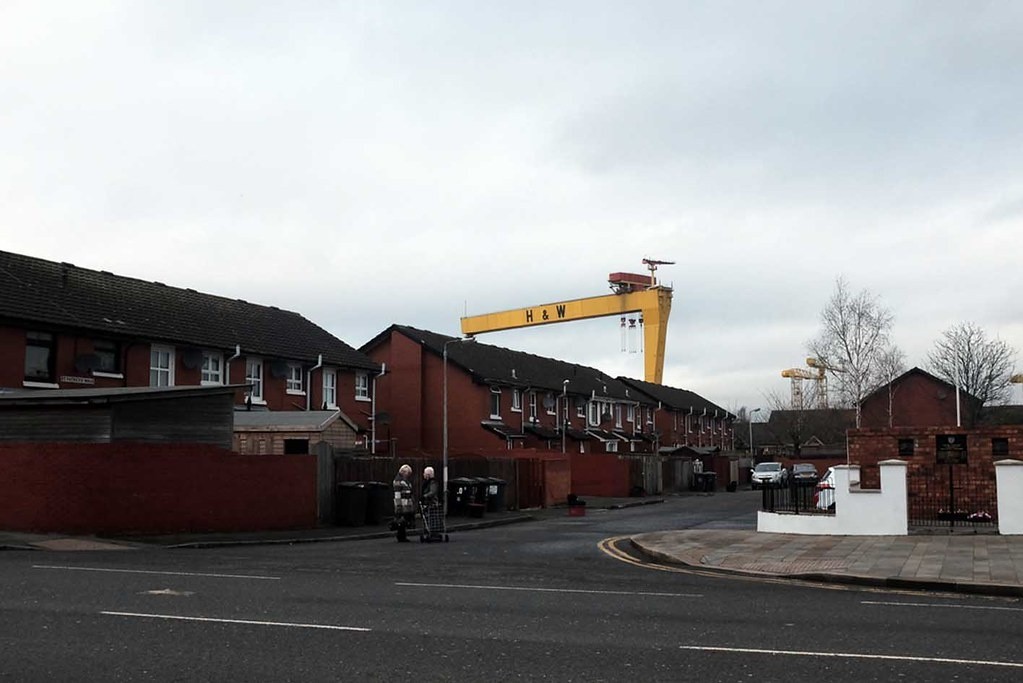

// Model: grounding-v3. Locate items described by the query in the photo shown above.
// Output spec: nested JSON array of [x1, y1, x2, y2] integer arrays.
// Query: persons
[[417, 466, 450, 542], [392, 464, 414, 543]]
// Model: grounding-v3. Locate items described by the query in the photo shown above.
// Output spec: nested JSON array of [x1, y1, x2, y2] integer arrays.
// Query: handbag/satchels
[[394, 496, 415, 515]]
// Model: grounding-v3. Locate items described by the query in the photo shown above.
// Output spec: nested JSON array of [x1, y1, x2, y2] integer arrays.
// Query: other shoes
[[396, 534, 409, 542]]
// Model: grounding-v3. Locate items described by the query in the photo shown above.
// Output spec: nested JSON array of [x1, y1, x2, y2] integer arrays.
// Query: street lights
[[749, 409, 761, 465], [443, 337, 477, 526]]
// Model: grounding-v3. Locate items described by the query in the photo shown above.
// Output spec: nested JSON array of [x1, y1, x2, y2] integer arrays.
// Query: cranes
[[781, 358, 847, 409]]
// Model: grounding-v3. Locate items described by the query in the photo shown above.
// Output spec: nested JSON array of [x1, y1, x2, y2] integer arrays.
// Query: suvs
[[751, 462, 788, 489], [813, 464, 862, 510]]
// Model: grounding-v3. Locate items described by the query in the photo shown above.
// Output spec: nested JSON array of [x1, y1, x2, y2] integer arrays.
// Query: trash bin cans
[[449, 478, 476, 517], [485, 476, 508, 513], [334, 480, 388, 528], [693, 471, 717, 493], [473, 477, 493, 513]]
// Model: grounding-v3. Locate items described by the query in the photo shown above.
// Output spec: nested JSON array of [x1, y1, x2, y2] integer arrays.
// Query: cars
[[790, 463, 819, 486]]
